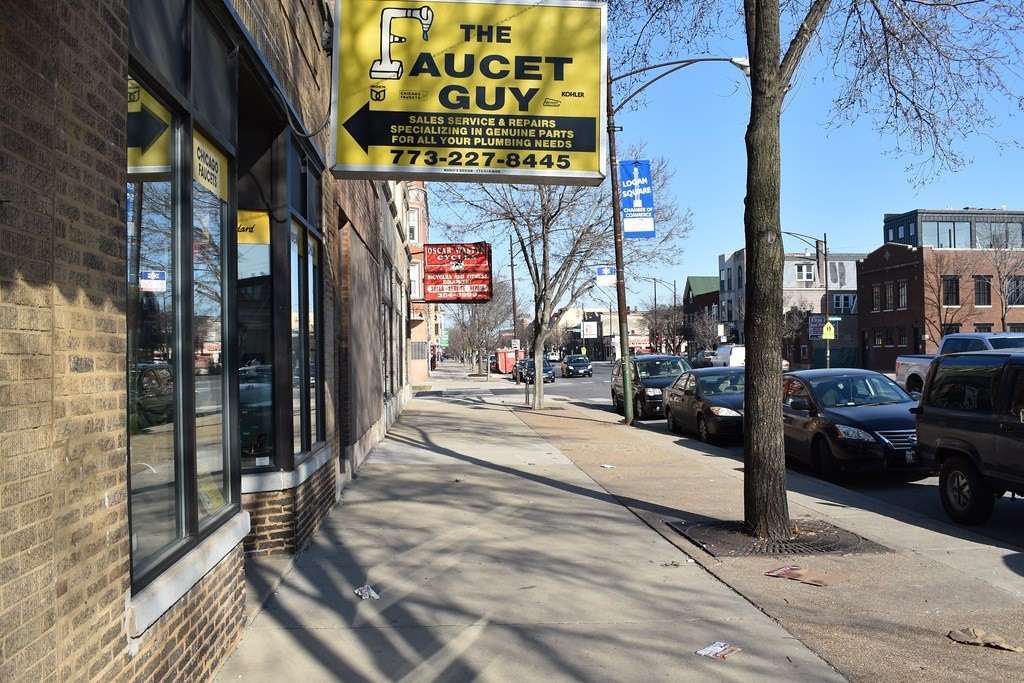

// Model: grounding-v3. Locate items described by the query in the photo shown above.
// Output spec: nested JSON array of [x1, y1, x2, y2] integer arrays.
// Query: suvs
[[915, 347, 1024, 525], [611, 355, 695, 421]]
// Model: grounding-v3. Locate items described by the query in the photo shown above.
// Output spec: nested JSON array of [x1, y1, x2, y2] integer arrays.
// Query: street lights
[[605, 54, 757, 423]]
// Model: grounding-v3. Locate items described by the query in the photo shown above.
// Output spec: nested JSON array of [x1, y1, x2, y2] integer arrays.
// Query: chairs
[[732, 375, 744, 391], [843, 384, 858, 402], [817, 382, 837, 405], [700, 379, 712, 393], [716, 377, 731, 392]]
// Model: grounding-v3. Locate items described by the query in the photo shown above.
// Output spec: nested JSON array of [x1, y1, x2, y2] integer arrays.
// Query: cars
[[560, 353, 593, 378], [523, 358, 556, 384], [782, 366, 930, 487], [473, 353, 494, 363], [781, 359, 790, 371], [512, 359, 526, 382], [195, 347, 316, 458], [662, 366, 746, 445], [691, 350, 718, 369], [137, 359, 178, 427]]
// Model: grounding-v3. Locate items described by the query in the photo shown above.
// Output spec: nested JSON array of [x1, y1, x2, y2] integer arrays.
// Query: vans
[[709, 342, 745, 367]]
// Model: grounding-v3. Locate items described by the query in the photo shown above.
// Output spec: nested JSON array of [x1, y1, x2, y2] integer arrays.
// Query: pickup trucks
[[894, 332, 1024, 397]]
